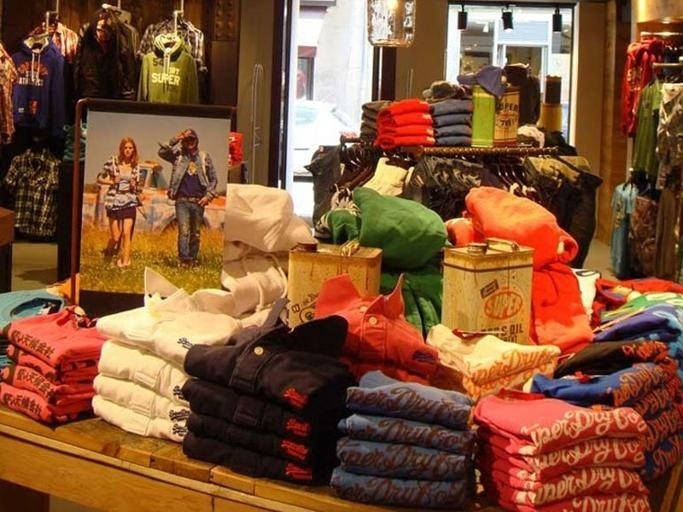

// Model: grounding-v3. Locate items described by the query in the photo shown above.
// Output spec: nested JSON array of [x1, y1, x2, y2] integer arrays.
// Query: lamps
[[367, 0, 417, 47], [447, 0, 576, 33]]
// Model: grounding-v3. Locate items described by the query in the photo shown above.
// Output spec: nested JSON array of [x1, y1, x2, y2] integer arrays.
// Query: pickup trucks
[[83, 156, 227, 233]]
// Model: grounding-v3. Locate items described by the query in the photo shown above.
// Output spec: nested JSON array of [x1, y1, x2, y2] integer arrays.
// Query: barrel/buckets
[[287, 239, 384, 329], [493, 86, 520, 147], [441, 237, 536, 348]]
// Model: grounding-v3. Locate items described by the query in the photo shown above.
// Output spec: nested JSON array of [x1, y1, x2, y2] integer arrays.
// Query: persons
[[156, 128, 219, 269], [93, 136, 141, 269]]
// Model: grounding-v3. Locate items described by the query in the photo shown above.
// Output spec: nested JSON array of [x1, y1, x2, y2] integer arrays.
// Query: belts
[[177, 198, 199, 203]]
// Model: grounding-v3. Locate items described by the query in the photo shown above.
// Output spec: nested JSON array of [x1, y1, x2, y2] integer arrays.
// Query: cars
[[280, 97, 361, 179]]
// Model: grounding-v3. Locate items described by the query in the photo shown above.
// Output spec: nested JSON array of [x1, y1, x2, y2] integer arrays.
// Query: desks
[[0, 403, 678, 511]]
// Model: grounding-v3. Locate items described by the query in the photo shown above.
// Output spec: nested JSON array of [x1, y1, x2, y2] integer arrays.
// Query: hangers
[[632, 33, 683, 86], [27, 138, 46, 161], [323, 137, 586, 200], [622, 167, 682, 201], [24, 7, 189, 61]]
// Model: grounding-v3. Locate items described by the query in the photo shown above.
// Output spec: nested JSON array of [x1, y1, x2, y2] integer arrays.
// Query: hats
[[182, 128, 198, 141], [422, 81, 457, 102], [456, 65, 510, 98]]
